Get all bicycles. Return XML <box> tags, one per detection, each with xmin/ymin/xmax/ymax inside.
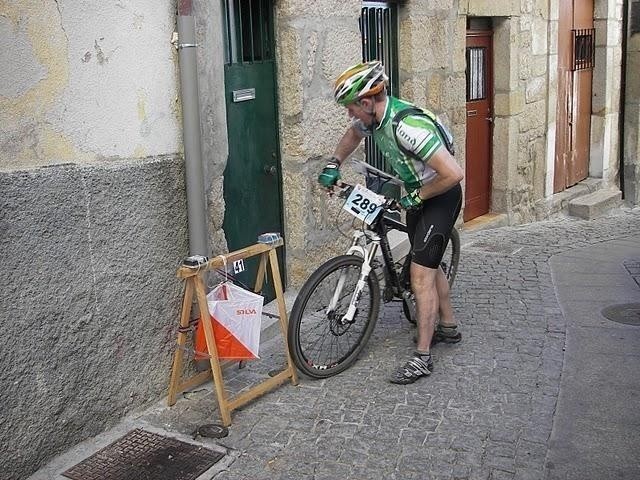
<box><xmin>284</xmin><ymin>172</ymin><xmax>466</xmax><ymax>375</ymax></box>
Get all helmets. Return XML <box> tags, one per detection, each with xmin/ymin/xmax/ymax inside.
<box><xmin>333</xmin><ymin>60</ymin><xmax>390</xmax><ymax>106</ymax></box>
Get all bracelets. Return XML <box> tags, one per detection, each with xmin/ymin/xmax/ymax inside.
<box><xmin>330</xmin><ymin>157</ymin><xmax>341</xmax><ymax>167</ymax></box>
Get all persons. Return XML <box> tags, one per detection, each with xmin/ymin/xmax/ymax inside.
<box><xmin>316</xmin><ymin>59</ymin><xmax>465</xmax><ymax>386</ymax></box>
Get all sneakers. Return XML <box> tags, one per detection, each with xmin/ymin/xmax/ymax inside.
<box><xmin>390</xmin><ymin>351</ymin><xmax>434</xmax><ymax>385</ymax></box>
<box><xmin>413</xmin><ymin>323</ymin><xmax>462</xmax><ymax>347</ymax></box>
